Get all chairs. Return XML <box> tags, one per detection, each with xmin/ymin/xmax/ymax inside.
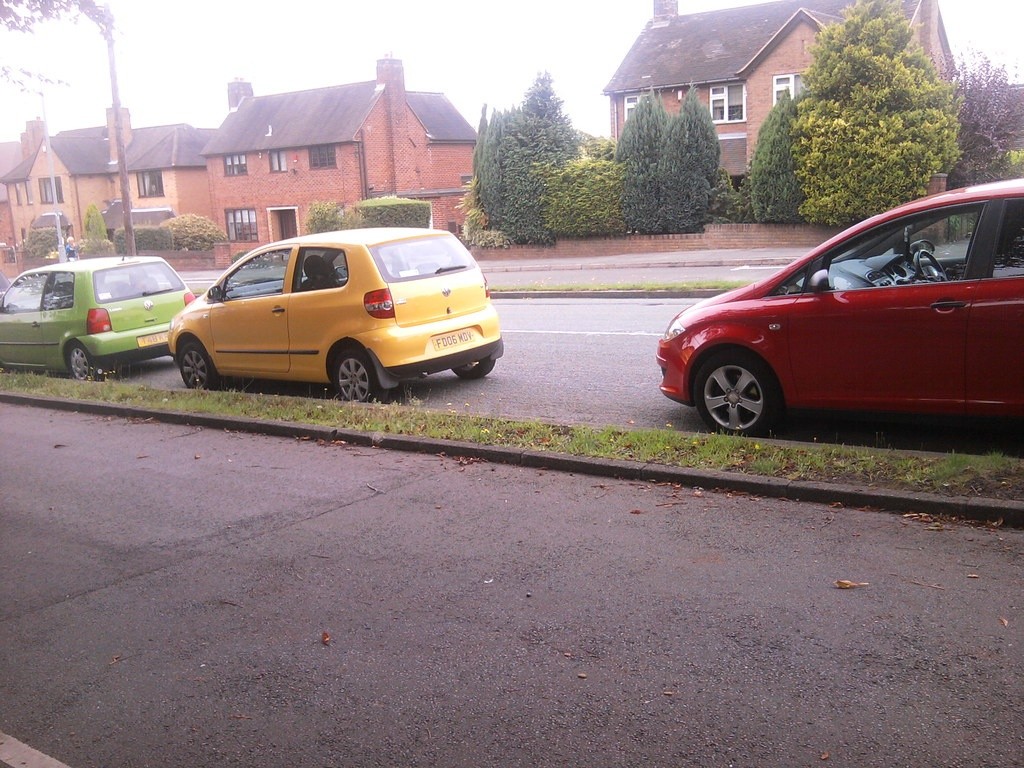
<box><xmin>302</xmin><ymin>255</ymin><xmax>333</xmax><ymax>291</ymax></box>
<box><xmin>51</xmin><ymin>281</ymin><xmax>74</xmax><ymax>309</ymax></box>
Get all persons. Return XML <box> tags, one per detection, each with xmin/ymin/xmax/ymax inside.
<box><xmin>65</xmin><ymin>236</ymin><xmax>80</xmax><ymax>263</ymax></box>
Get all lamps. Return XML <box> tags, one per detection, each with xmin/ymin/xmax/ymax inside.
<box><xmin>290</xmin><ymin>169</ymin><xmax>297</xmax><ymax>175</ymax></box>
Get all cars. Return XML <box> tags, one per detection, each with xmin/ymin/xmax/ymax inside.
<box><xmin>656</xmin><ymin>175</ymin><xmax>1024</xmax><ymax>434</ymax></box>
<box><xmin>0</xmin><ymin>254</ymin><xmax>195</xmax><ymax>379</ymax></box>
<box><xmin>166</xmin><ymin>227</ymin><xmax>504</xmax><ymax>403</ymax></box>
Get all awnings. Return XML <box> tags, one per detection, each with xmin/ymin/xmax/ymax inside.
<box><xmin>32</xmin><ymin>212</ymin><xmax>73</xmax><ymax>228</ymax></box>
<box><xmin>90</xmin><ymin>199</ymin><xmax>176</xmax><ymax>230</ymax></box>
